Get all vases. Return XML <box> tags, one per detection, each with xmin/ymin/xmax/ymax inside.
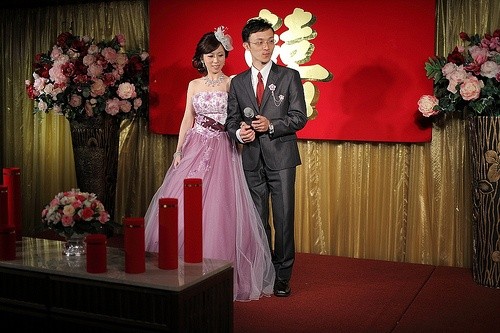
<box><xmin>467</xmin><ymin>112</ymin><xmax>500</xmax><ymax>289</ymax></box>
<box><xmin>68</xmin><ymin>112</ymin><xmax>119</xmax><ymax>221</ymax></box>
<box><xmin>59</xmin><ymin>231</ymin><xmax>88</xmax><ymax>256</ymax></box>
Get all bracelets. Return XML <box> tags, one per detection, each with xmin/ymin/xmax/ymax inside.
<box><xmin>174</xmin><ymin>151</ymin><xmax>182</xmax><ymax>157</ymax></box>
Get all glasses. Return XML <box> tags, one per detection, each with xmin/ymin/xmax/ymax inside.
<box><xmin>248</xmin><ymin>38</ymin><xmax>276</xmax><ymax>46</ymax></box>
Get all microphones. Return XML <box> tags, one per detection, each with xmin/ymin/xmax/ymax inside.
<box><xmin>243</xmin><ymin>107</ymin><xmax>257</xmax><ymax>121</ymax></box>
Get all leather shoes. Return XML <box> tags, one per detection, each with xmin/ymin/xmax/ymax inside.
<box><xmin>274</xmin><ymin>283</ymin><xmax>291</xmax><ymax>297</ymax></box>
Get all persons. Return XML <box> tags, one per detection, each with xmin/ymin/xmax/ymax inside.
<box><xmin>227</xmin><ymin>19</ymin><xmax>306</xmax><ymax>296</ymax></box>
<box><xmin>142</xmin><ymin>27</ymin><xmax>277</xmax><ymax>303</ymax></box>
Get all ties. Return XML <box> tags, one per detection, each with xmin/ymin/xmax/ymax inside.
<box><xmin>256</xmin><ymin>72</ymin><xmax>264</xmax><ymax>109</ymax></box>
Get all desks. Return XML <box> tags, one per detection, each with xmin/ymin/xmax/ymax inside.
<box><xmin>0</xmin><ymin>237</ymin><xmax>234</xmax><ymax>333</ymax></box>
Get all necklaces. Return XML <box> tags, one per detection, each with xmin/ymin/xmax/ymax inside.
<box><xmin>203</xmin><ymin>71</ymin><xmax>224</xmax><ymax>87</ymax></box>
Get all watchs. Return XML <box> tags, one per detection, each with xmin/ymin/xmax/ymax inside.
<box><xmin>268</xmin><ymin>122</ymin><xmax>274</xmax><ymax>134</ymax></box>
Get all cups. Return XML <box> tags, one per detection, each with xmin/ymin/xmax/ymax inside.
<box><xmin>0</xmin><ymin>167</ymin><xmax>22</xmax><ymax>261</ymax></box>
<box><xmin>184</xmin><ymin>178</ymin><xmax>202</xmax><ymax>263</ymax></box>
<box><xmin>86</xmin><ymin>234</ymin><xmax>108</xmax><ymax>273</ymax></box>
<box><xmin>125</xmin><ymin>217</ymin><xmax>146</xmax><ymax>273</ymax></box>
<box><xmin>158</xmin><ymin>198</ymin><xmax>178</xmax><ymax>269</ymax></box>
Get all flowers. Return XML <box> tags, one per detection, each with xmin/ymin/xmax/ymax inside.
<box><xmin>25</xmin><ymin>32</ymin><xmax>148</xmax><ymax>123</ymax></box>
<box><xmin>417</xmin><ymin>29</ymin><xmax>500</xmax><ymax>120</ymax></box>
<box><xmin>41</xmin><ymin>189</ymin><xmax>114</xmax><ymax>238</ymax></box>
